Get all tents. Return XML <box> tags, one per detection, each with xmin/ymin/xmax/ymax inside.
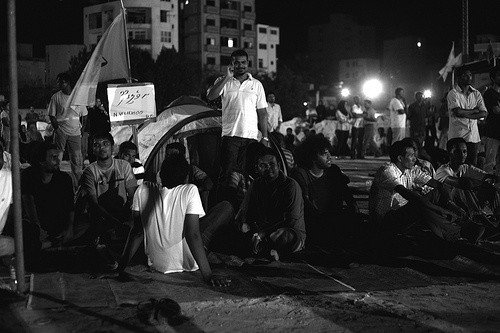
<box><xmin>133</xmin><ymin>106</ymin><xmax>222</xmax><ymax>183</ymax></box>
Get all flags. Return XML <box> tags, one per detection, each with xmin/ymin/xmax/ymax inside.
<box><xmin>65</xmin><ymin>9</ymin><xmax>129</xmax><ymax>117</ymax></box>
<box><xmin>438</xmin><ymin>46</ymin><xmax>462</xmax><ymax>84</ymax></box>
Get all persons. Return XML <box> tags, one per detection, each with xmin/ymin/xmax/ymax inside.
<box><xmin>207</xmin><ymin>50</ymin><xmax>269</xmax><ymax>204</ymax></box>
<box><xmin>0</xmin><ymin>67</ymin><xmax>500</xmax><ymax>288</ymax></box>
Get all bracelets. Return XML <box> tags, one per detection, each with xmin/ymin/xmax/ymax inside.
<box><xmin>261</xmin><ymin>137</ymin><xmax>269</xmax><ymax>142</ymax></box>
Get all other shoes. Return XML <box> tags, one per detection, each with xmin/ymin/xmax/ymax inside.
<box><xmin>136</xmin><ymin>297</ymin><xmax>187</xmax><ymax>326</ymax></box>
